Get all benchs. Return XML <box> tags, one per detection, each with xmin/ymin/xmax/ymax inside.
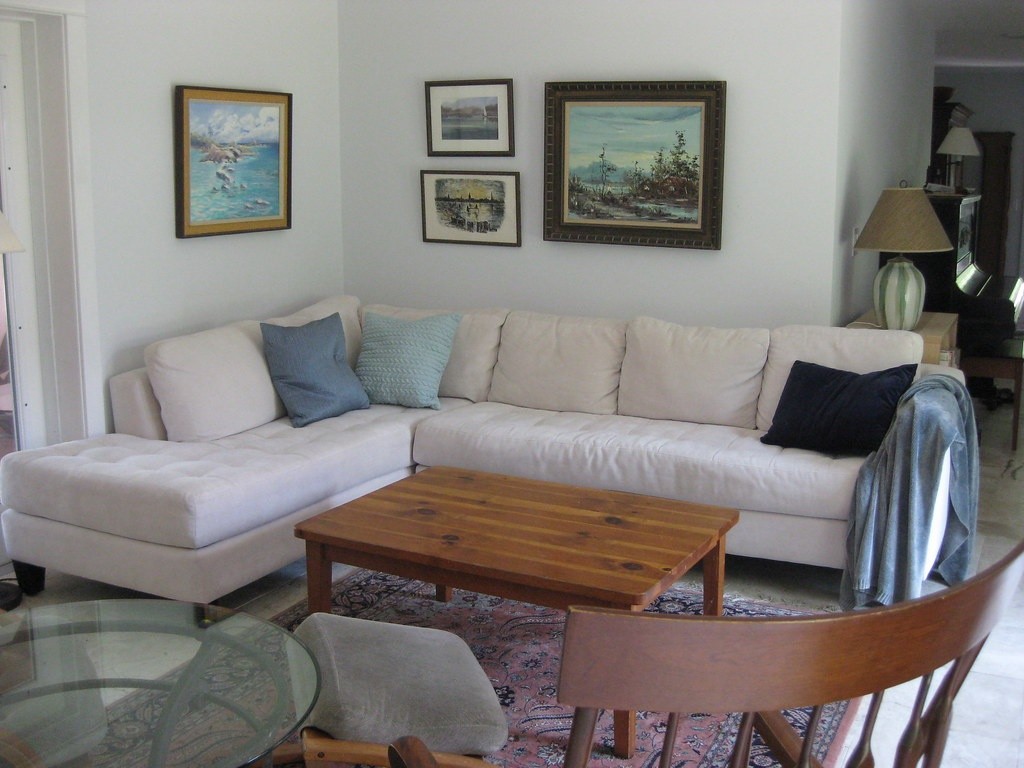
<box><xmin>961</xmin><ymin>331</ymin><xmax>1024</xmax><ymax>450</ymax></box>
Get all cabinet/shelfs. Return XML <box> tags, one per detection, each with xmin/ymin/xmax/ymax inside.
<box><xmin>849</xmin><ymin>308</ymin><xmax>958</xmax><ymax>365</ymax></box>
<box><xmin>936</xmin><ymin>103</ymin><xmax>976</xmax><ymax>193</ymax></box>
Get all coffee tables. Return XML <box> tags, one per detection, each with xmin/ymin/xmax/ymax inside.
<box><xmin>295</xmin><ymin>465</ymin><xmax>739</xmax><ymax>758</ymax></box>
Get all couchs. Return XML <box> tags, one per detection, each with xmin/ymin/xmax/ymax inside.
<box><xmin>2</xmin><ymin>295</ymin><xmax>967</xmax><ymax>626</ymax></box>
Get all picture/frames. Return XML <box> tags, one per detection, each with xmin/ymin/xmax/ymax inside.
<box><xmin>543</xmin><ymin>81</ymin><xmax>727</xmax><ymax>250</ymax></box>
<box><xmin>420</xmin><ymin>170</ymin><xmax>521</xmax><ymax>247</ymax></box>
<box><xmin>175</xmin><ymin>85</ymin><xmax>293</xmax><ymax>238</ymax></box>
<box><xmin>425</xmin><ymin>78</ymin><xmax>515</xmax><ymax>157</ymax></box>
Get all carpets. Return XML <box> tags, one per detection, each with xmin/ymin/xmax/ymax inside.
<box><xmin>0</xmin><ymin>569</ymin><xmax>860</xmax><ymax>768</ymax></box>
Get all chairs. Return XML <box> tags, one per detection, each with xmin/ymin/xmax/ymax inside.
<box><xmin>287</xmin><ymin>612</ymin><xmax>508</xmax><ymax>768</ymax></box>
<box><xmin>556</xmin><ymin>538</ymin><xmax>1024</xmax><ymax>768</ymax></box>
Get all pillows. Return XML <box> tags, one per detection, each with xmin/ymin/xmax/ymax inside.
<box><xmin>260</xmin><ymin>312</ymin><xmax>370</xmax><ymax>427</ymax></box>
<box><xmin>356</xmin><ymin>311</ymin><xmax>461</xmax><ymax>410</ymax></box>
<box><xmin>760</xmin><ymin>360</ymin><xmax>918</xmax><ymax>457</ymax></box>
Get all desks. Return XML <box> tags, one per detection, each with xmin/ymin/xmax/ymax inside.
<box><xmin>0</xmin><ymin>600</ymin><xmax>321</xmax><ymax>768</ymax></box>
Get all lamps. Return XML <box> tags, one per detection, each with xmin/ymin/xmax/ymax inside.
<box><xmin>930</xmin><ymin>126</ymin><xmax>981</xmax><ymax>170</ymax></box>
<box><xmin>854</xmin><ymin>180</ymin><xmax>954</xmax><ymax>331</ymax></box>
